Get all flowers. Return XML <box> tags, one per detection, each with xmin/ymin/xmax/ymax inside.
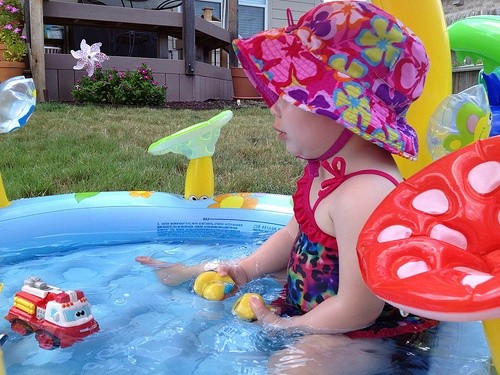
<box><xmin>0</xmin><ymin>0</ymin><xmax>28</xmax><ymax>62</ymax></box>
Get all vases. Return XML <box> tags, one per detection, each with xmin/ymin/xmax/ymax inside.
<box><xmin>231</xmin><ymin>68</ymin><xmax>257</xmax><ymax>97</ymax></box>
<box><xmin>0</xmin><ymin>44</ymin><xmax>25</xmax><ymax>81</ymax></box>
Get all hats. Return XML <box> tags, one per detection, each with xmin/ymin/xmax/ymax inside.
<box><xmin>231</xmin><ymin>0</ymin><xmax>430</xmax><ymax>164</ymax></box>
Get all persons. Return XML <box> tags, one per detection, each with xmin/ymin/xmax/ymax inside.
<box><xmin>131</xmin><ymin>1</ymin><xmax>442</xmax><ymax>375</ymax></box>
